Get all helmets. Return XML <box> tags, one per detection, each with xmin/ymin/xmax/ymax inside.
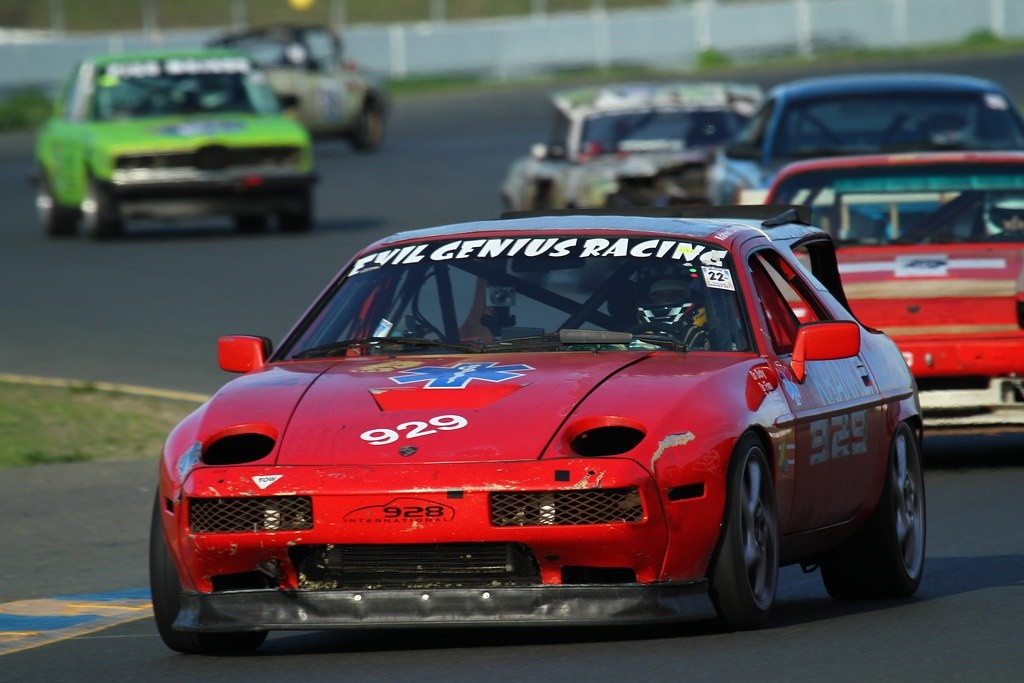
<box><xmin>927</xmin><ymin>101</ymin><xmax>970</xmax><ymax>147</ymax></box>
<box><xmin>981</xmin><ymin>189</ymin><xmax>1024</xmax><ymax>237</ymax></box>
<box><xmin>632</xmin><ymin>257</ymin><xmax>711</xmax><ymax>333</ymax></box>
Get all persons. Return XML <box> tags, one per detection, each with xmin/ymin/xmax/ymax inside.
<box><xmin>623</xmin><ymin>273</ymin><xmax>716</xmax><ymax>351</ymax></box>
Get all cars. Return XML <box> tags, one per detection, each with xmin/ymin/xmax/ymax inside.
<box><xmin>206</xmin><ymin>23</ymin><xmax>388</xmax><ymax>150</ymax></box>
<box><xmin>704</xmin><ymin>75</ymin><xmax>1024</xmax><ymax>206</ymax></box>
<box><xmin>36</xmin><ymin>47</ymin><xmax>318</xmax><ymax>237</ymax></box>
<box><xmin>767</xmin><ymin>147</ymin><xmax>1024</xmax><ymax>437</ymax></box>
<box><xmin>498</xmin><ymin>81</ymin><xmax>766</xmax><ymax>219</ymax></box>
<box><xmin>149</xmin><ymin>212</ymin><xmax>931</xmax><ymax>649</ymax></box>
